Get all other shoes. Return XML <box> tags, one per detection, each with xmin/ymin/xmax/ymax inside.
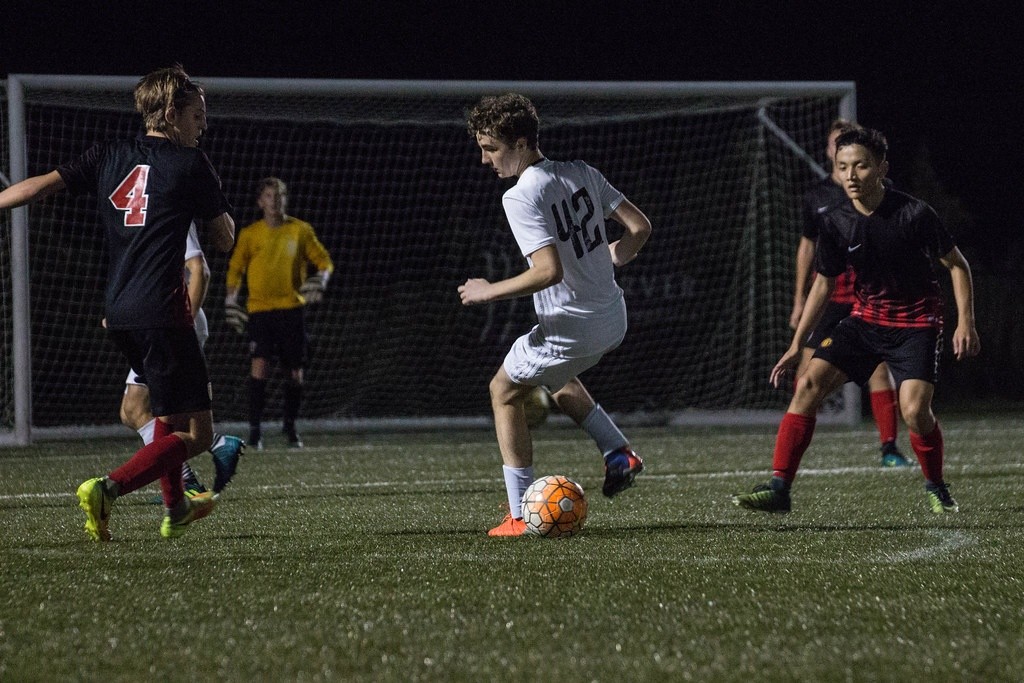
<box><xmin>247</xmin><ymin>437</ymin><xmax>262</xmax><ymax>451</ymax></box>
<box><xmin>283</xmin><ymin>426</ymin><xmax>304</xmax><ymax>448</ymax></box>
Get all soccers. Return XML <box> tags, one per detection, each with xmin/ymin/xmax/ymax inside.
<box><xmin>520</xmin><ymin>475</ymin><xmax>588</xmax><ymax>538</ymax></box>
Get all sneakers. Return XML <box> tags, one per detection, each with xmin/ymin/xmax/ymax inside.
<box><xmin>488</xmin><ymin>513</ymin><xmax>527</xmax><ymax>536</ymax></box>
<box><xmin>603</xmin><ymin>449</ymin><xmax>643</xmax><ymax>498</ymax></box>
<box><xmin>156</xmin><ymin>480</ymin><xmax>206</xmax><ymax>504</ymax></box>
<box><xmin>731</xmin><ymin>480</ymin><xmax>790</xmax><ymax>514</ymax></box>
<box><xmin>881</xmin><ymin>443</ymin><xmax>916</xmax><ymax>467</ymax></box>
<box><xmin>161</xmin><ymin>492</ymin><xmax>219</xmax><ymax>538</ymax></box>
<box><xmin>76</xmin><ymin>478</ymin><xmax>114</xmax><ymax>542</ymax></box>
<box><xmin>923</xmin><ymin>481</ymin><xmax>959</xmax><ymax>514</ymax></box>
<box><xmin>212</xmin><ymin>435</ymin><xmax>245</xmax><ymax>494</ymax></box>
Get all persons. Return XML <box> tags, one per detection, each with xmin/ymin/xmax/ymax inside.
<box><xmin>121</xmin><ymin>222</ymin><xmax>244</xmax><ymax>504</ymax></box>
<box><xmin>0</xmin><ymin>66</ymin><xmax>235</xmax><ymax>543</ymax></box>
<box><xmin>732</xmin><ymin>118</ymin><xmax>979</xmax><ymax>514</ymax></box>
<box><xmin>458</xmin><ymin>92</ymin><xmax>651</xmax><ymax>537</ymax></box>
<box><xmin>225</xmin><ymin>178</ymin><xmax>334</xmax><ymax>449</ymax></box>
